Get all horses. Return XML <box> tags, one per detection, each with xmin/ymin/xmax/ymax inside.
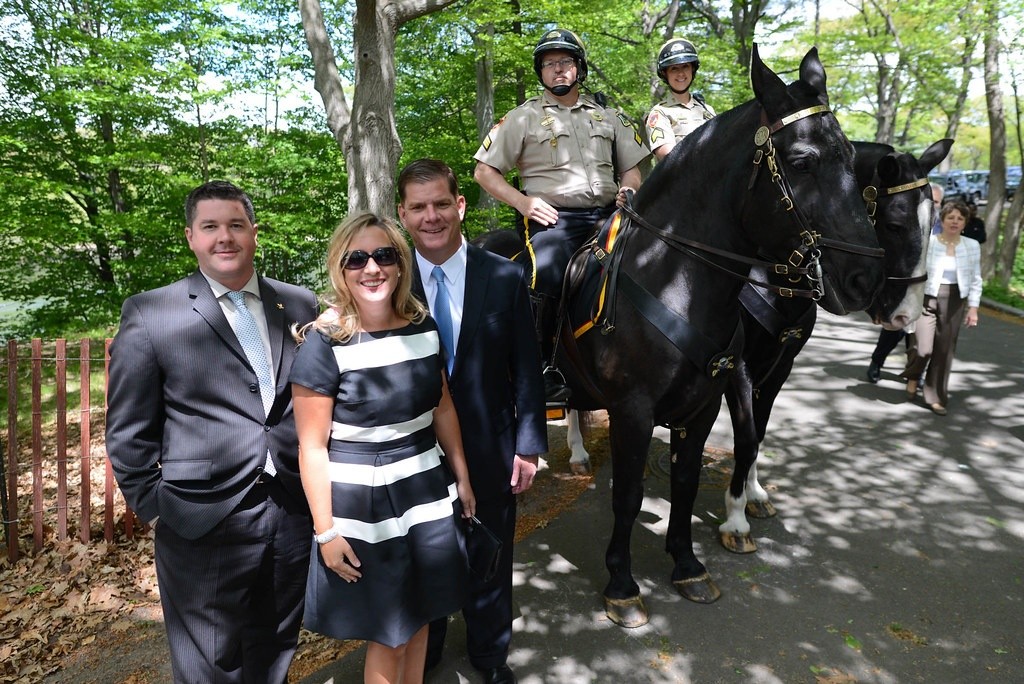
<box><xmin>471</xmin><ymin>41</ymin><xmax>955</xmax><ymax>629</ymax></box>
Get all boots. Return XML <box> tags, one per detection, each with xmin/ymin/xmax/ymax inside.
<box><xmin>528</xmin><ymin>286</ymin><xmax>571</xmax><ymax>400</ymax></box>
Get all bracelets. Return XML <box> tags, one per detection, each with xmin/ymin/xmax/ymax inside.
<box><xmin>313</xmin><ymin>525</ymin><xmax>338</xmax><ymax>544</ymax></box>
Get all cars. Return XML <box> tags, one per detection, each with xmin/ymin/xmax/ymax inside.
<box><xmin>951</xmin><ymin>169</ymin><xmax>990</xmax><ymax>204</ymax></box>
<box><xmin>1005</xmin><ymin>167</ymin><xmax>1022</xmax><ymax>199</ymax></box>
<box><xmin>927</xmin><ymin>173</ymin><xmax>964</xmax><ymax>207</ymax></box>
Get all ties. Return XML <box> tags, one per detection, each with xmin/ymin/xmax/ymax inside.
<box><xmin>226</xmin><ymin>291</ymin><xmax>278</xmax><ymax>476</ymax></box>
<box><xmin>432</xmin><ymin>266</ymin><xmax>456</xmax><ymax>380</ymax></box>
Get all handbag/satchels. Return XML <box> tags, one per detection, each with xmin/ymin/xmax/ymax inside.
<box><xmin>453</xmin><ymin>504</ymin><xmax>502</xmax><ymax>582</ymax></box>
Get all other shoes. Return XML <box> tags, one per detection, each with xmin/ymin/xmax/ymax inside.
<box><xmin>868</xmin><ymin>360</ymin><xmax>879</xmax><ymax>382</ymax></box>
<box><xmin>906</xmin><ymin>379</ymin><xmax>918</xmax><ymax>399</ymax></box>
<box><xmin>927</xmin><ymin>402</ymin><xmax>947</xmax><ymax>415</ymax></box>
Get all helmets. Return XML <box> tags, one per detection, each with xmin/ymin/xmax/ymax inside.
<box><xmin>532</xmin><ymin>28</ymin><xmax>589</xmax><ymax>77</ymax></box>
<box><xmin>657</xmin><ymin>41</ymin><xmax>700</xmax><ymax>78</ymax></box>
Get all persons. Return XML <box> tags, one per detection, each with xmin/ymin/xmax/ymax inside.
<box><xmin>473</xmin><ymin>29</ymin><xmax>649</xmax><ymax>402</ymax></box>
<box><xmin>105</xmin><ymin>180</ymin><xmax>319</xmax><ymax>684</ymax></box>
<box><xmin>897</xmin><ymin>198</ymin><xmax>987</xmax><ymax>416</ymax></box>
<box><xmin>645</xmin><ymin>38</ymin><xmax>717</xmax><ymax>160</ymax></box>
<box><xmin>867</xmin><ymin>182</ymin><xmax>944</xmax><ymax>388</ymax></box>
<box><xmin>287</xmin><ymin>214</ymin><xmax>475</xmax><ymax>684</ymax></box>
<box><xmin>397</xmin><ymin>159</ymin><xmax>548</xmax><ymax>684</ymax></box>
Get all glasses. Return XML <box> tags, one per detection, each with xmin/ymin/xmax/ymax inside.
<box><xmin>539</xmin><ymin>57</ymin><xmax>576</xmax><ymax>68</ymax></box>
<box><xmin>341</xmin><ymin>247</ymin><xmax>399</xmax><ymax>270</ymax></box>
